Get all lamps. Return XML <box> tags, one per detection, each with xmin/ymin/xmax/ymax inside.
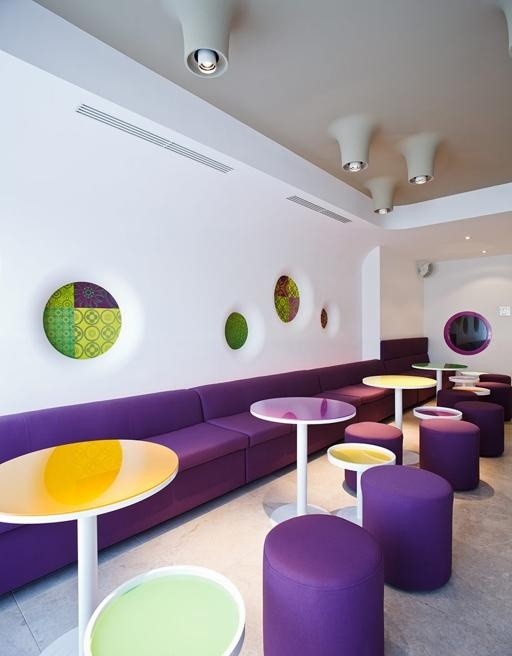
<box><xmin>415</xmin><ymin>176</ymin><xmax>427</xmax><ymax>184</ymax></box>
<box><xmin>349</xmin><ymin>162</ymin><xmax>362</xmax><ymax>173</ymax></box>
<box><xmin>379</xmin><ymin>208</ymin><xmax>387</xmax><ymax>215</ymax></box>
<box><xmin>197</xmin><ymin>50</ymin><xmax>217</xmax><ymax>74</ymax></box>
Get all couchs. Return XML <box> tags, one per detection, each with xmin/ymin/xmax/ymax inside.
<box><xmin>191</xmin><ymin>370</ymin><xmax>362</xmax><ymax>485</ymax></box>
<box><xmin>0</xmin><ymin>389</ymin><xmax>250</xmax><ymax>597</ymax></box>
<box><xmin>381</xmin><ymin>337</ymin><xmax>455</xmax><ymax>406</ymax></box>
<box><xmin>314</xmin><ymin>359</ymin><xmax>419</xmax><ymax>421</ymax></box>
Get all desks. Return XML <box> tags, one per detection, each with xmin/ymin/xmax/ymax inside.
<box><xmin>363</xmin><ymin>375</ymin><xmax>439</xmax><ymax>467</ymax></box>
<box><xmin>0</xmin><ymin>439</ymin><xmax>180</xmax><ymax>656</ymax></box>
<box><xmin>413</xmin><ymin>405</ymin><xmax>463</xmax><ymax>421</ymax></box>
<box><xmin>412</xmin><ymin>363</ymin><xmax>468</xmax><ymax>393</ymax></box>
<box><xmin>83</xmin><ymin>564</ymin><xmax>246</xmax><ymax>656</ymax></box>
<box><xmin>327</xmin><ymin>442</ymin><xmax>396</xmax><ymax>526</ymax></box>
<box><xmin>449</xmin><ymin>376</ymin><xmax>477</xmax><ymax>386</ymax></box>
<box><xmin>251</xmin><ymin>397</ymin><xmax>357</xmax><ymax>528</ymax></box>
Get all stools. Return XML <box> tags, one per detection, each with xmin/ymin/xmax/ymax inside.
<box><xmin>476</xmin><ymin>382</ymin><xmax>512</xmax><ymax>421</ymax></box>
<box><xmin>479</xmin><ymin>374</ymin><xmax>511</xmax><ymax>384</ymax></box>
<box><xmin>455</xmin><ymin>400</ymin><xmax>504</xmax><ymax>458</ymax></box>
<box><xmin>437</xmin><ymin>390</ymin><xmax>479</xmax><ymax>409</ymax></box>
<box><xmin>361</xmin><ymin>465</ymin><xmax>454</xmax><ymax>591</ymax></box>
<box><xmin>263</xmin><ymin>513</ymin><xmax>385</xmax><ymax>655</ymax></box>
<box><xmin>419</xmin><ymin>418</ymin><xmax>481</xmax><ymax>490</ymax></box>
<box><xmin>345</xmin><ymin>422</ymin><xmax>403</xmax><ymax>491</ymax></box>
